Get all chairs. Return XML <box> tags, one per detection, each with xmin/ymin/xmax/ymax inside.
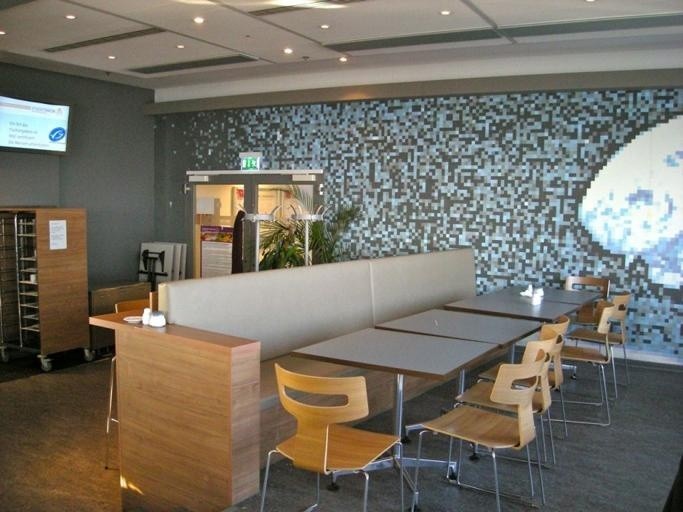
<box><xmin>104</xmin><ymin>299</ymin><xmax>150</xmax><ymax>470</ymax></box>
<box><xmin>261</xmin><ymin>276</ymin><xmax>632</xmax><ymax>512</ymax></box>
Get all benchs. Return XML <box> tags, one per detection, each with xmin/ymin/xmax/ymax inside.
<box><xmin>156</xmin><ymin>247</ymin><xmax>505</xmax><ymax>469</ymax></box>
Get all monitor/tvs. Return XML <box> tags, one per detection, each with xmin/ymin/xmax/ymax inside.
<box><xmin>0</xmin><ymin>91</ymin><xmax>74</xmax><ymax>156</ymax></box>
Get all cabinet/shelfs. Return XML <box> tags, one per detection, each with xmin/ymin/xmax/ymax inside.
<box><xmin>0</xmin><ymin>205</ymin><xmax>93</xmax><ymax>371</ymax></box>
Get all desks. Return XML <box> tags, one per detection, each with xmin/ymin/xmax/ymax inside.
<box><xmin>260</xmin><ymin>275</ymin><xmax>633</xmax><ymax>511</ymax></box>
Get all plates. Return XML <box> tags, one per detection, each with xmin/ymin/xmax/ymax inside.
<box><xmin>122</xmin><ymin>316</ymin><xmax>142</xmax><ymax>324</ymax></box>
